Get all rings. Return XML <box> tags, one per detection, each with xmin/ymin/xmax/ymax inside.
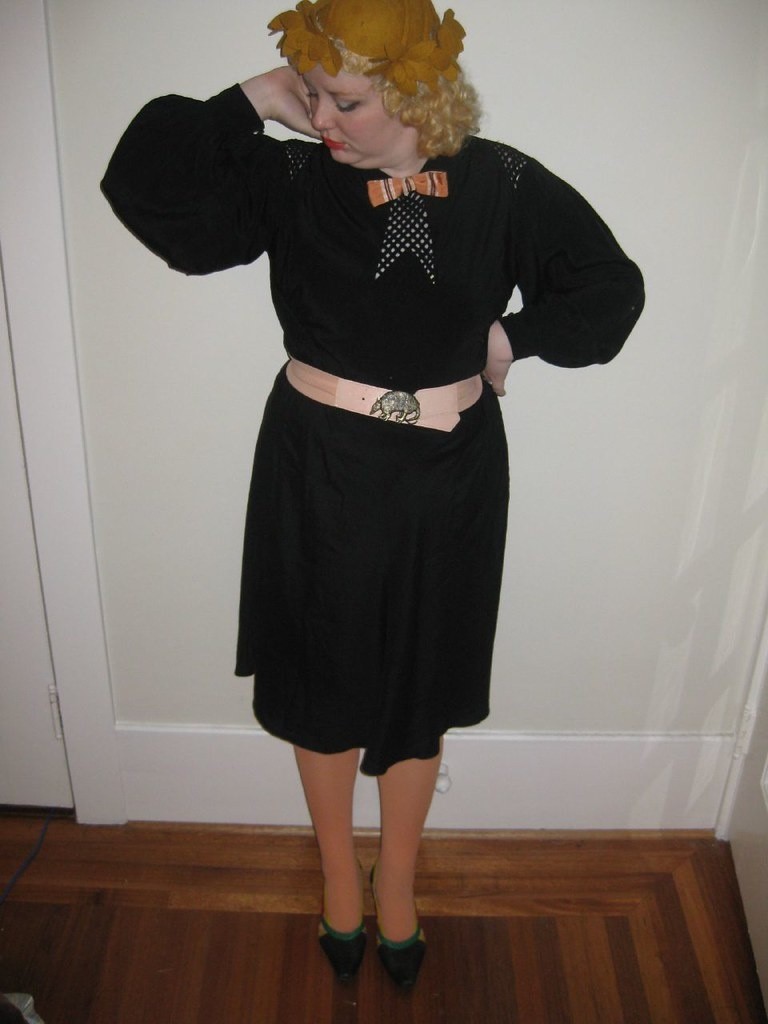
<box><xmin>487</xmin><ymin>381</ymin><xmax>492</xmax><ymax>384</ymax></box>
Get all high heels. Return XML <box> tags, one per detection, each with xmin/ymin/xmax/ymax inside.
<box><xmin>370</xmin><ymin>861</ymin><xmax>425</xmax><ymax>995</ymax></box>
<box><xmin>318</xmin><ymin>858</ymin><xmax>367</xmax><ymax>986</ymax></box>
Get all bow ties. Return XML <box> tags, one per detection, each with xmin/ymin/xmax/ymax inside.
<box><xmin>366</xmin><ymin>170</ymin><xmax>449</xmax><ymax>286</ymax></box>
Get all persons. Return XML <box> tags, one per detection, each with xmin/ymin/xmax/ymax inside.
<box><xmin>96</xmin><ymin>0</ymin><xmax>646</xmax><ymax>988</ymax></box>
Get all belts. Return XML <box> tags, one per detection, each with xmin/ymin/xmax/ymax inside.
<box><xmin>285</xmin><ymin>351</ymin><xmax>483</xmax><ymax>432</ymax></box>
